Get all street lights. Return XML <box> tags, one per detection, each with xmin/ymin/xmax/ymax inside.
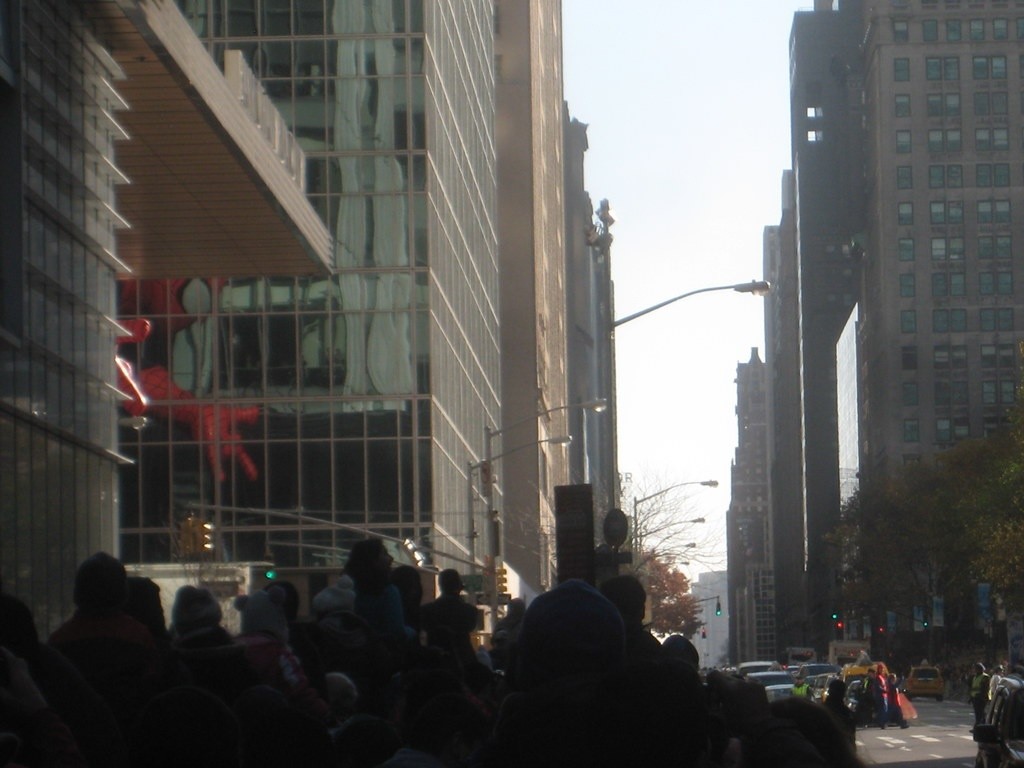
<box><xmin>633</xmin><ymin>477</ymin><xmax>720</xmax><ymax>581</ymax></box>
<box><xmin>481</xmin><ymin>396</ymin><xmax>607</xmax><ymax>622</ymax></box>
<box><xmin>604</xmin><ymin>202</ymin><xmax>770</xmax><ymax>506</ymax></box>
<box><xmin>466</xmin><ymin>435</ymin><xmax>572</xmax><ymax>607</ymax></box>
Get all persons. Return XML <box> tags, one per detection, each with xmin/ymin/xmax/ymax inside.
<box><xmin>987</xmin><ymin>664</ymin><xmax>1005</xmax><ymax>700</ymax></box>
<box><xmin>1</xmin><ymin>533</ymin><xmax>862</xmax><ymax>767</ymax></box>
<box><xmin>872</xmin><ymin>664</ymin><xmax>890</xmax><ymax>727</ymax></box>
<box><xmin>966</xmin><ymin>661</ymin><xmax>990</xmax><ymax>733</ymax></box>
<box><xmin>854</xmin><ymin>668</ymin><xmax>877</xmax><ymax>724</ymax></box>
<box><xmin>881</xmin><ymin>673</ymin><xmax>910</xmax><ymax>729</ymax></box>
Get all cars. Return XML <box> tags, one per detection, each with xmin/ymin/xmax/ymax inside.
<box><xmin>716</xmin><ymin>639</ymin><xmax>899</xmax><ymax>726</ymax></box>
<box><xmin>972</xmin><ymin>665</ymin><xmax>1024</xmax><ymax>768</ymax></box>
<box><xmin>904</xmin><ymin>666</ymin><xmax>945</xmax><ymax>701</ymax></box>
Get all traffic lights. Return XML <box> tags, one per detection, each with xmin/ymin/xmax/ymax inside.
<box><xmin>878</xmin><ymin>625</ymin><xmax>884</xmax><ymax>633</ymax></box>
<box><xmin>832</xmin><ymin>608</ymin><xmax>843</xmax><ymax>631</ymax></box>
<box><xmin>264</xmin><ymin>555</ymin><xmax>276</xmax><ymax>579</ymax></box>
<box><xmin>716</xmin><ymin>603</ymin><xmax>720</xmax><ymax>616</ymax></box>
<box><xmin>923</xmin><ymin>616</ymin><xmax>928</xmax><ymax>628</ymax></box>
<box><xmin>702</xmin><ymin>628</ymin><xmax>707</xmax><ymax>639</ymax></box>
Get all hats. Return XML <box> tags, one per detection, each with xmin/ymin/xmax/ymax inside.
<box><xmin>795</xmin><ymin>674</ymin><xmax>804</xmax><ymax>680</ymax></box>
<box><xmin>265</xmin><ymin>582</ymin><xmax>300</xmax><ymax>606</ymax></box>
<box><xmin>438</xmin><ymin>569</ymin><xmax>464</xmax><ymax>586</ymax></box>
<box><xmin>126</xmin><ymin>578</ymin><xmax>163</xmax><ymax>614</ymax></box>
<box><xmin>74</xmin><ymin>553</ymin><xmax>129</xmax><ymax>604</ymax></box>
<box><xmin>868</xmin><ymin>668</ymin><xmax>874</xmax><ymax>672</ymax></box>
<box><xmin>601</xmin><ymin>573</ymin><xmax>646</xmax><ymax>602</ymax></box>
<box><xmin>520</xmin><ymin>578</ymin><xmax>625</xmax><ymax>666</ymax></box>
<box><xmin>977</xmin><ymin>662</ymin><xmax>985</xmax><ymax>671</ymax></box>
